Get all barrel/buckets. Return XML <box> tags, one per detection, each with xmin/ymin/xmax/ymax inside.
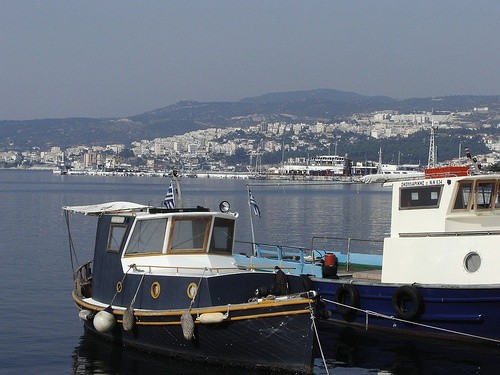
<box><xmin>324</xmin><ymin>253</ymin><xmax>335</xmax><ymax>267</ymax></box>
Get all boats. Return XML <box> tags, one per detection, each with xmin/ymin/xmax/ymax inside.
<box><xmin>228</xmin><ymin>165</ymin><xmax>500</xmax><ymax>346</ymax></box>
<box><xmin>61</xmin><ymin>200</ymin><xmax>320</xmax><ymax>375</ymax></box>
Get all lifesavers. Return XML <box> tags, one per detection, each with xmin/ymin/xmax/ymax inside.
<box><xmin>392</xmin><ymin>286</ymin><xmax>424</xmax><ymax>321</ymax></box>
<box><xmin>336</xmin><ymin>284</ymin><xmax>361</xmax><ymax>322</ymax></box>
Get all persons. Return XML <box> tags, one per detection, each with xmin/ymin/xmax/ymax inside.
<box><xmin>273</xmin><ymin>266</ymin><xmax>289</xmax><ymax>296</ymax></box>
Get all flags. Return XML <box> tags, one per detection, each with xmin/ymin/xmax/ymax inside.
<box><xmin>248</xmin><ymin>189</ymin><xmax>261</xmax><ymax>217</ymax></box>
<box><xmin>163</xmin><ymin>181</ymin><xmax>175</xmax><ymax>210</ymax></box>
